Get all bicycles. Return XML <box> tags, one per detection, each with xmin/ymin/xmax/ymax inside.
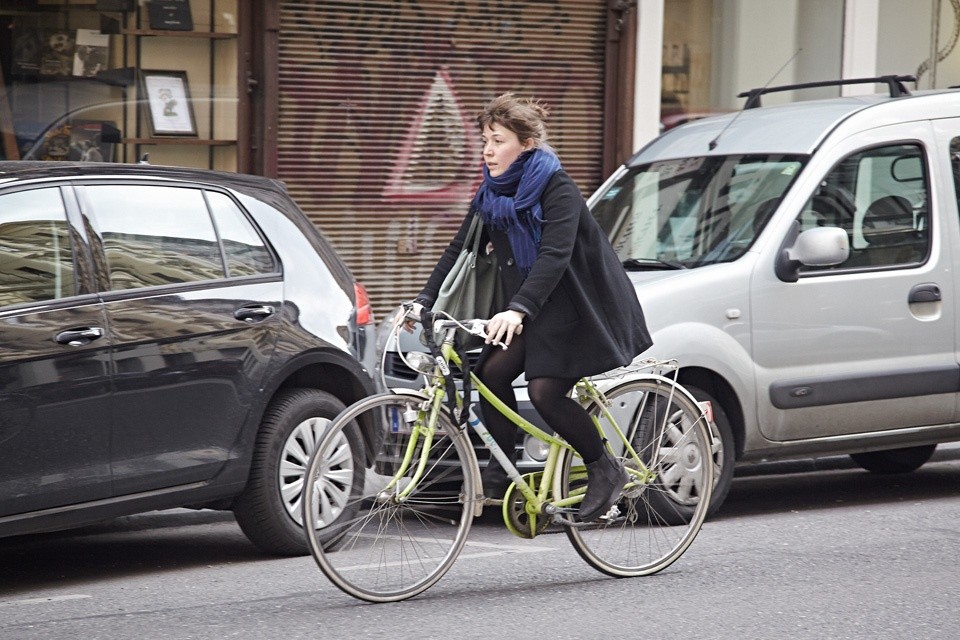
<box><xmin>301</xmin><ymin>308</ymin><xmax>724</xmax><ymax>604</ymax></box>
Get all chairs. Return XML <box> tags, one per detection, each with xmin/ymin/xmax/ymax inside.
<box><xmin>752</xmin><ymin>197</ymin><xmax>803</xmax><ymax>240</ymax></box>
<box><xmin>859</xmin><ymin>196</ymin><xmax>927</xmax><ymax>266</ymax></box>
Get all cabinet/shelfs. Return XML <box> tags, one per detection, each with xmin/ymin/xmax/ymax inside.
<box><xmin>0</xmin><ymin>0</ymin><xmax>241</xmax><ymax>172</ymax></box>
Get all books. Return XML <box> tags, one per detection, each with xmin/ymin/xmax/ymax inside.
<box><xmin>7</xmin><ymin>27</ymin><xmax>110</xmax><ymax>77</ymax></box>
<box><xmin>14</xmin><ymin>120</ymin><xmax>116</xmax><ymax>162</ymax></box>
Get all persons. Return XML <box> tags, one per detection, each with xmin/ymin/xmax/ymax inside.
<box><xmin>395</xmin><ymin>90</ymin><xmax>653</xmax><ymax>522</ymax></box>
<box><xmin>78</xmin><ymin>45</ymin><xmax>102</xmax><ymax>77</ymax></box>
<box><xmin>75</xmin><ymin>139</ymin><xmax>103</xmax><ymax>162</ymax></box>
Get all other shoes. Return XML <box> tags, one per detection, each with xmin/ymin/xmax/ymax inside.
<box><xmin>579</xmin><ymin>450</ymin><xmax>629</xmax><ymax>524</ymax></box>
<box><xmin>461</xmin><ymin>449</ymin><xmax>518</xmax><ymax>499</ymax></box>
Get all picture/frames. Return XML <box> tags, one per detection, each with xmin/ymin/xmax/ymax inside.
<box><xmin>136</xmin><ymin>67</ymin><xmax>199</xmax><ymax>139</ymax></box>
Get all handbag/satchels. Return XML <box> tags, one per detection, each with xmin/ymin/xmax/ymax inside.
<box><xmin>431</xmin><ymin>206</ymin><xmax>511</xmax><ymax>353</ymax></box>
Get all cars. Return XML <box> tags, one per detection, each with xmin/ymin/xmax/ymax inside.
<box><xmin>0</xmin><ymin>152</ymin><xmax>387</xmax><ymax>557</ymax></box>
<box><xmin>368</xmin><ymin>75</ymin><xmax>959</xmax><ymax>519</ymax></box>
